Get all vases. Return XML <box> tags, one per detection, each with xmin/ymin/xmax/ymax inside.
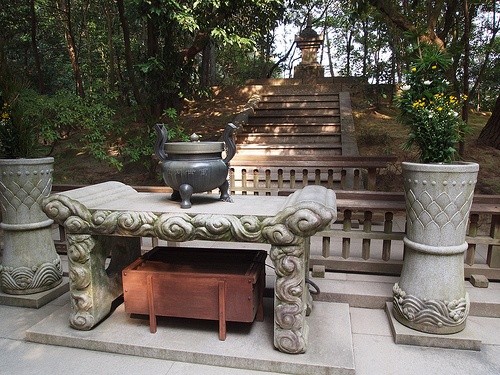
<box><xmin>392</xmin><ymin>160</ymin><xmax>479</xmax><ymax>335</ymax></box>
<box><xmin>0</xmin><ymin>157</ymin><xmax>62</xmax><ymax>296</ymax></box>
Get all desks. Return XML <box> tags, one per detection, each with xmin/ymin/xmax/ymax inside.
<box><xmin>40</xmin><ymin>180</ymin><xmax>337</xmax><ymax>354</ymax></box>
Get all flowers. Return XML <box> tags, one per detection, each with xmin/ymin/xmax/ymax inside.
<box><xmin>392</xmin><ymin>25</ymin><xmax>477</xmax><ymax>165</ymax></box>
<box><xmin>0</xmin><ymin>76</ymin><xmax>46</xmax><ymax>159</ymax></box>
